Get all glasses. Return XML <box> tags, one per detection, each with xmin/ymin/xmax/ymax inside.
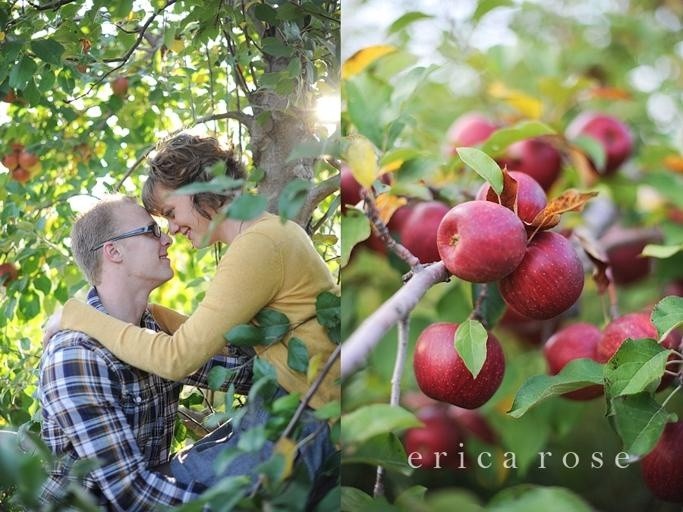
<box><xmin>92</xmin><ymin>225</ymin><xmax>162</xmax><ymax>251</ymax></box>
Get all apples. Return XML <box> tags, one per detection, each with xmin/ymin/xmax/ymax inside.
<box><xmin>371</xmin><ymin>171</ymin><xmax>393</xmax><ymax>192</ymax></box>
<box><xmin>74</xmin><ymin>143</ymin><xmax>90</xmax><ymax>160</ymax></box>
<box><xmin>0</xmin><ymin>264</ymin><xmax>18</xmax><ymax>285</ymax></box>
<box><xmin>364</xmin><ymin>231</ymin><xmax>386</xmax><ymax>253</ymax></box>
<box><xmin>76</xmin><ymin>60</ymin><xmax>87</xmax><ymax>73</ymax></box>
<box><xmin>340</xmin><ymin>167</ymin><xmax>361</xmax><ymax>205</ymax></box>
<box><xmin>10</xmin><ymin>143</ymin><xmax>23</xmax><ymax>155</ymax></box>
<box><xmin>571</xmin><ymin>113</ymin><xmax>633</xmax><ymax>177</ymax></box>
<box><xmin>15</xmin><ymin>94</ymin><xmax>28</xmax><ymax>106</ymax></box>
<box><xmin>642</xmin><ymin>420</ymin><xmax>683</xmax><ymax>500</ymax></box>
<box><xmin>609</xmin><ymin>243</ymin><xmax>651</xmax><ymax>286</ymax></box>
<box><xmin>13</xmin><ymin>165</ymin><xmax>29</xmax><ymax>182</ymax></box>
<box><xmin>402</xmin><ymin>390</ymin><xmax>442</xmax><ymax>418</ymax></box>
<box><xmin>3</xmin><ymin>88</ymin><xmax>15</xmax><ymax>102</ymax></box>
<box><xmin>79</xmin><ymin>39</ymin><xmax>91</xmax><ymax>52</ymax></box>
<box><xmin>436</xmin><ymin>201</ymin><xmax>527</xmax><ymax>284</ymax></box>
<box><xmin>544</xmin><ymin>323</ymin><xmax>606</xmax><ymax>402</ymax></box>
<box><xmin>661</xmin><ymin>278</ymin><xmax>682</xmax><ymax>301</ymax></box>
<box><xmin>499</xmin><ymin>230</ymin><xmax>585</xmax><ymax>322</ymax></box>
<box><xmin>645</xmin><ymin>303</ymin><xmax>680</xmax><ymax>392</ymax></box>
<box><xmin>404</xmin><ymin>404</ymin><xmax>463</xmax><ymax>471</ymax></box>
<box><xmin>18</xmin><ymin>149</ymin><xmax>40</xmax><ymax>169</ymax></box>
<box><xmin>414</xmin><ymin>321</ymin><xmax>506</xmax><ymax>409</ymax></box>
<box><xmin>374</xmin><ymin>191</ymin><xmax>410</xmax><ymax>232</ymax></box>
<box><xmin>110</xmin><ymin>77</ymin><xmax>128</xmax><ymax>94</ymax></box>
<box><xmin>400</xmin><ymin>200</ymin><xmax>450</xmax><ymax>265</ymax></box>
<box><xmin>477</xmin><ymin>170</ymin><xmax>549</xmax><ymax>239</ymax></box>
<box><xmin>2</xmin><ymin>154</ymin><xmax>20</xmax><ymax>171</ymax></box>
<box><xmin>444</xmin><ymin>113</ymin><xmax>495</xmax><ymax>158</ymax></box>
<box><xmin>450</xmin><ymin>404</ymin><xmax>499</xmax><ymax>446</ymax></box>
<box><xmin>597</xmin><ymin>313</ymin><xmax>657</xmax><ymax>365</ymax></box>
<box><xmin>499</xmin><ymin>140</ymin><xmax>562</xmax><ymax>189</ymax></box>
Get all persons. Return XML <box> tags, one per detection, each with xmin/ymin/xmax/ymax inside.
<box><xmin>41</xmin><ymin>199</ymin><xmax>255</xmax><ymax>511</ymax></box>
<box><xmin>41</xmin><ymin>134</ymin><xmax>340</xmax><ymax>509</ymax></box>
